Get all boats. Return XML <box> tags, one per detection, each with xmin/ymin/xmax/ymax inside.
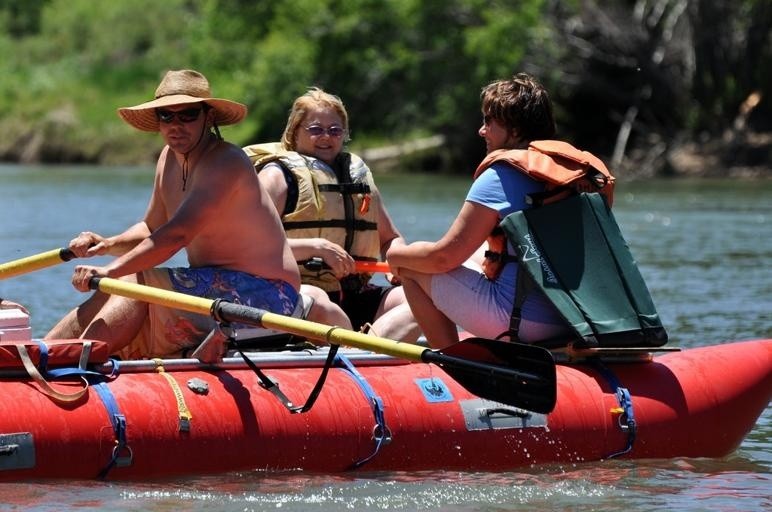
<box><xmin>0</xmin><ymin>260</ymin><xmax>770</xmax><ymax>487</ymax></box>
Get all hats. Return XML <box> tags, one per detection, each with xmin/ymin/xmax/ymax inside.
<box><xmin>116</xmin><ymin>69</ymin><xmax>248</xmax><ymax>132</ymax></box>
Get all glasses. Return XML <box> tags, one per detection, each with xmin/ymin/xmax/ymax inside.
<box><xmin>483</xmin><ymin>114</ymin><xmax>500</xmax><ymax>127</ymax></box>
<box><xmin>155</xmin><ymin>104</ymin><xmax>203</xmax><ymax>124</ymax></box>
<box><xmin>298</xmin><ymin>122</ymin><xmax>346</xmax><ymax>138</ymax></box>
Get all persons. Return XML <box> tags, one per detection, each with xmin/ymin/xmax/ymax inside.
<box><xmin>41</xmin><ymin>69</ymin><xmax>302</xmax><ymax>361</ymax></box>
<box><xmin>254</xmin><ymin>86</ymin><xmax>423</xmax><ymax>354</ymax></box>
<box><xmin>385</xmin><ymin>71</ymin><xmax>616</xmax><ymax>350</ymax></box>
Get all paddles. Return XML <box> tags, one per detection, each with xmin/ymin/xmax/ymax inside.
<box><xmin>89</xmin><ymin>276</ymin><xmax>556</xmax><ymax>414</ymax></box>
